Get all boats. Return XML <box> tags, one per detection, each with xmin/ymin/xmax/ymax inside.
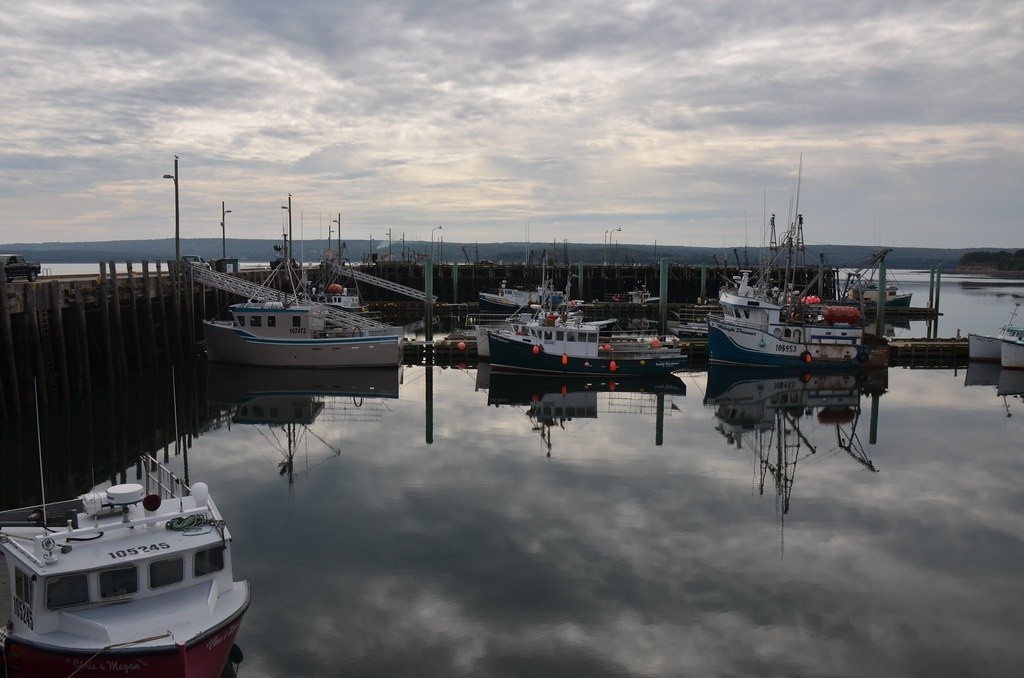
<box><xmin>202</xmin><ymin>214</ymin><xmax>404</xmax><ymax>369</ymax></box>
<box><xmin>1</xmin><ymin>366</ymin><xmax>250</xmax><ymax>678</ymax></box>
<box><xmin>963</xmin><ymin>362</ymin><xmax>1024</xmax><ymax>419</ymax></box>
<box><xmin>474</xmin><ymin>152</ymin><xmax>912</xmax><ymax>378</ymax></box>
<box><xmin>968</xmin><ymin>302</ymin><xmax>1024</xmax><ymax>370</ymax></box>
<box><xmin>191</xmin><ymin>352</ymin><xmax>404</xmax><ymax>503</ymax></box>
<box><xmin>703</xmin><ymin>365</ymin><xmax>880</xmax><ymax>561</ymax></box>
<box><xmin>475</xmin><ymin>360</ymin><xmax>687</xmax><ymax>458</ymax></box>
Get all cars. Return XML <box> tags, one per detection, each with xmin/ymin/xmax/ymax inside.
<box><xmin>480</xmin><ymin>260</ymin><xmax>494</xmax><ymax>267</ymax></box>
<box><xmin>656</xmin><ymin>258</ymin><xmax>680</xmax><ymax>267</ymax></box>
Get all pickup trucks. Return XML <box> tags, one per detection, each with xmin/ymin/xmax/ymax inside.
<box><xmin>172</xmin><ymin>255</ymin><xmax>210</xmax><ymax>275</ymax></box>
<box><xmin>0</xmin><ymin>254</ymin><xmax>41</xmax><ymax>282</ymax></box>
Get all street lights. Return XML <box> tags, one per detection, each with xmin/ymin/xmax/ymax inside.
<box><xmin>386</xmin><ymin>227</ymin><xmax>391</xmax><ymax>261</ymax></box>
<box><xmin>329</xmin><ymin>225</ymin><xmax>335</xmax><ymax>250</ymax></box>
<box><xmin>333</xmin><ymin>213</ymin><xmax>341</xmax><ymax>265</ymax></box>
<box><xmin>370</xmin><ymin>234</ymin><xmax>374</xmax><ymax>254</ymax></box>
<box><xmin>162</xmin><ymin>159</ymin><xmax>180</xmax><ymax>281</ymax></box>
<box><xmin>425</xmin><ymin>225</ymin><xmax>443</xmax><ymax>304</ymax></box>
<box><xmin>221</xmin><ymin>202</ymin><xmax>232</xmax><ymax>258</ymax></box>
<box><xmin>605</xmin><ymin>227</ymin><xmax>621</xmax><ymax>265</ymax></box>
<box><xmin>400</xmin><ymin>231</ymin><xmax>404</xmax><ymax>262</ymax></box>
<box><xmin>281</xmin><ymin>197</ymin><xmax>292</xmax><ymax>269</ymax></box>
